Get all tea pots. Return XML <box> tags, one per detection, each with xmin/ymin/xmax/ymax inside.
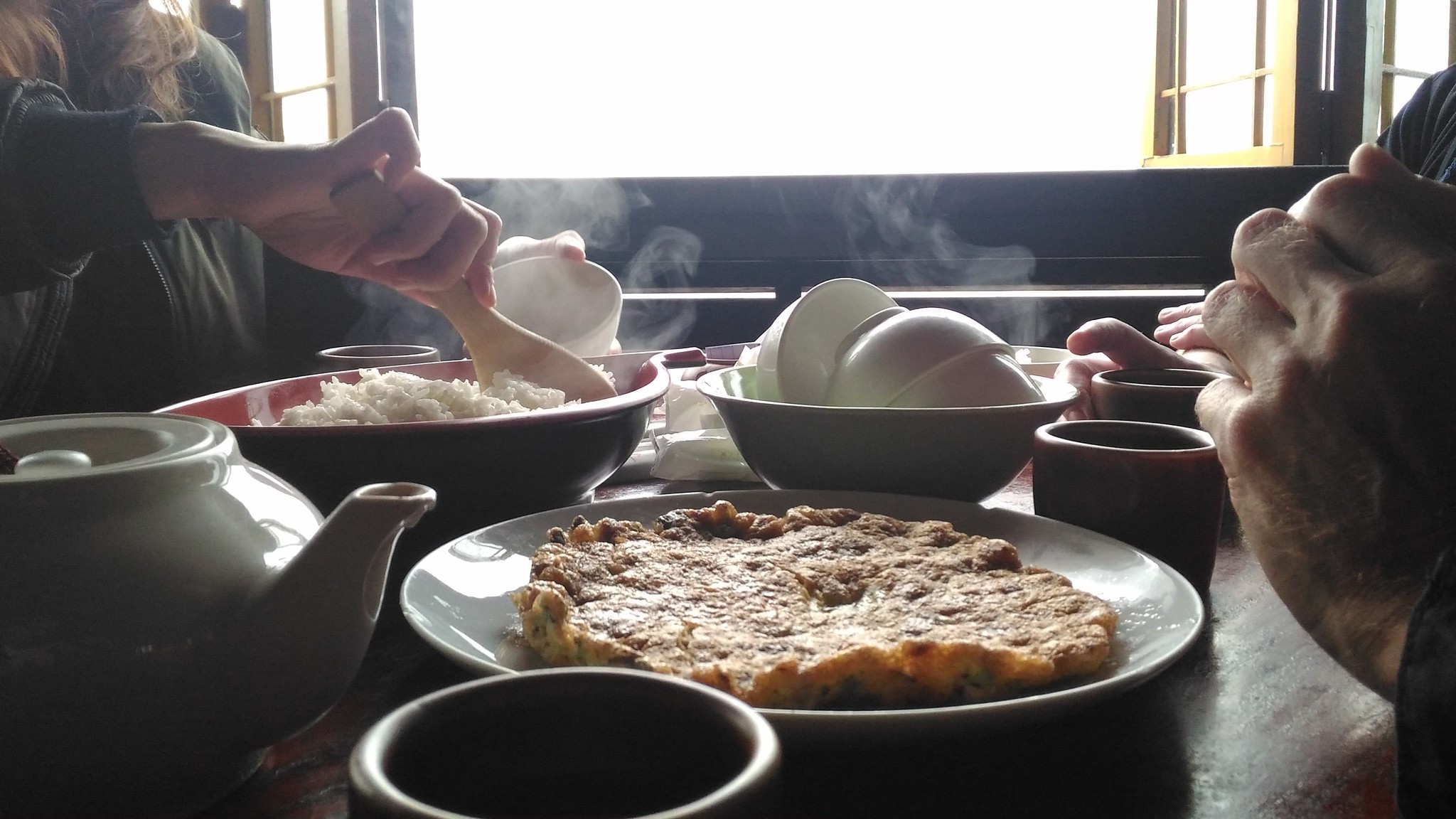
<box><xmin>1</xmin><ymin>410</ymin><xmax>437</xmax><ymax>819</ymax></box>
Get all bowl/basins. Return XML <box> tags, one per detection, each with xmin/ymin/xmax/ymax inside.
<box><xmin>827</xmin><ymin>306</ymin><xmax>1047</xmax><ymax>407</ymax></box>
<box><xmin>493</xmin><ymin>255</ymin><xmax>623</xmax><ymax>358</ymax></box>
<box><xmin>149</xmin><ymin>347</ymin><xmax>707</xmax><ymax>548</ymax></box>
<box><xmin>1012</xmin><ymin>345</ymin><xmax>1070</xmax><ymax>379</ymax></box>
<box><xmin>756</xmin><ymin>277</ymin><xmax>898</xmax><ymax>407</ymax></box>
<box><xmin>696</xmin><ymin>365</ymin><xmax>1082</xmax><ymax>505</ymax></box>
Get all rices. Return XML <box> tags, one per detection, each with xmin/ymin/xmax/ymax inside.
<box><xmin>249</xmin><ymin>360</ymin><xmax>616</xmax><ymax>425</ymax></box>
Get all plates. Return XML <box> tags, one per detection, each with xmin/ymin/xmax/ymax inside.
<box><xmin>399</xmin><ymin>490</ymin><xmax>1205</xmax><ymax>738</ymax></box>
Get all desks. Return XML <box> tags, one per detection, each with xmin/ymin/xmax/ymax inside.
<box><xmin>181</xmin><ymin>459</ymin><xmax>1400</xmax><ymax>819</ymax></box>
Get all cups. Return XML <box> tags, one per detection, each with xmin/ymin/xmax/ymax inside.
<box><xmin>1091</xmin><ymin>368</ymin><xmax>1233</xmax><ymax>431</ymax></box>
<box><xmin>1032</xmin><ymin>420</ymin><xmax>1227</xmax><ymax>593</ymax></box>
<box><xmin>309</xmin><ymin>345</ymin><xmax>440</xmax><ymax>375</ymax></box>
<box><xmin>349</xmin><ymin>666</ymin><xmax>783</xmax><ymax>819</ymax></box>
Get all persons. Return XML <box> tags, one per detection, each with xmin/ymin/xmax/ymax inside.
<box><xmin>0</xmin><ymin>0</ymin><xmax>502</xmax><ymax>410</ymax></box>
<box><xmin>1051</xmin><ymin>58</ymin><xmax>1456</xmax><ymax>819</ymax></box>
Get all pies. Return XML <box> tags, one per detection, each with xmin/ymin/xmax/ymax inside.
<box><xmin>518</xmin><ymin>496</ymin><xmax>1119</xmax><ymax>708</ymax></box>
